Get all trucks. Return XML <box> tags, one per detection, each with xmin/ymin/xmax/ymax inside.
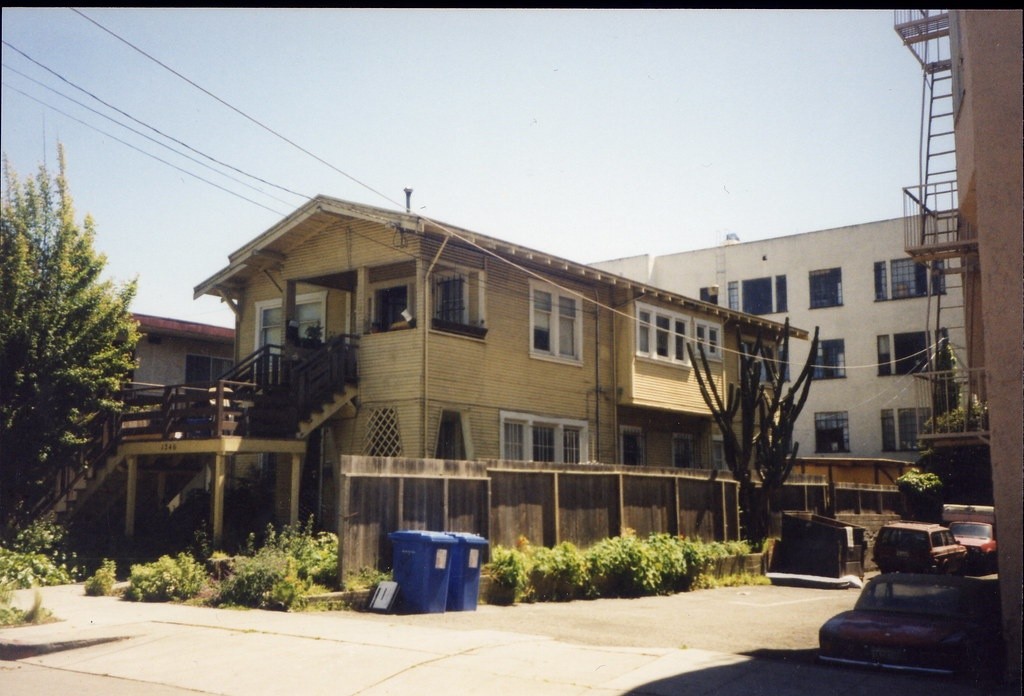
<box><xmin>941</xmin><ymin>505</ymin><xmax>997</xmax><ymax>570</ymax></box>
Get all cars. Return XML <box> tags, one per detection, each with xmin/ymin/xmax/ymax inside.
<box><xmin>816</xmin><ymin>572</ymin><xmax>1004</xmax><ymax>678</ymax></box>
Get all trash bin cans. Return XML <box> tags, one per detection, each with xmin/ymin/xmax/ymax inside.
<box><xmin>445</xmin><ymin>532</ymin><xmax>489</xmax><ymax>612</ymax></box>
<box><xmin>782</xmin><ymin>510</ymin><xmax>867</xmax><ymax>582</ymax></box>
<box><xmin>388</xmin><ymin>530</ymin><xmax>459</xmax><ymax>614</ymax></box>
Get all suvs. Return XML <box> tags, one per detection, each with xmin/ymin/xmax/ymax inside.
<box><xmin>872</xmin><ymin>522</ymin><xmax>969</xmax><ymax>578</ymax></box>
